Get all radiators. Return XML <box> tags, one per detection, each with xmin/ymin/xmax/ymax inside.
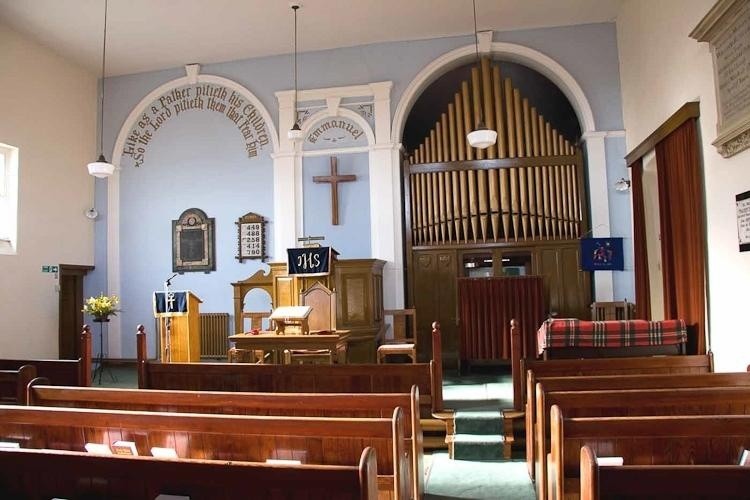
<box><xmin>200</xmin><ymin>313</ymin><xmax>228</xmax><ymax>360</ymax></box>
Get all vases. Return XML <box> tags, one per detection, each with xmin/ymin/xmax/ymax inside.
<box><xmin>94</xmin><ymin>311</ymin><xmax>108</xmax><ymax>321</ymax></box>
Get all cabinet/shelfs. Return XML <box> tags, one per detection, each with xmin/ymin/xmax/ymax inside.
<box><xmin>266</xmin><ymin>258</ymin><xmax>388</xmax><ymax>365</ymax></box>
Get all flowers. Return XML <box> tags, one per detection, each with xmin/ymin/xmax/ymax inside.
<box><xmin>80</xmin><ymin>291</ymin><xmax>119</xmax><ymax>314</ymax></box>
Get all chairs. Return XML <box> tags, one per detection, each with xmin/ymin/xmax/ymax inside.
<box><xmin>375</xmin><ymin>305</ymin><xmax>418</xmax><ymax>364</ymax></box>
<box><xmin>228</xmin><ymin>309</ymin><xmax>273</xmax><ymax>364</ymax></box>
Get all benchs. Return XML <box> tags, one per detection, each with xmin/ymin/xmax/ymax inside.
<box><xmin>517</xmin><ymin>348</ymin><xmax>750</xmax><ymax>500</ymax></box>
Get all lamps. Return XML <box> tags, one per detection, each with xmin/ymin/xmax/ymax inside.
<box><xmin>286</xmin><ymin>0</ymin><xmax>307</xmax><ymax>140</ymax></box>
<box><xmin>86</xmin><ymin>0</ymin><xmax>114</xmax><ymax>180</ymax></box>
<box><xmin>465</xmin><ymin>1</ymin><xmax>498</xmax><ymax>150</ymax></box>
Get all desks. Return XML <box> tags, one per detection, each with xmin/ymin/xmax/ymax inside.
<box><xmin>228</xmin><ymin>329</ymin><xmax>352</xmax><ymax>365</ymax></box>
